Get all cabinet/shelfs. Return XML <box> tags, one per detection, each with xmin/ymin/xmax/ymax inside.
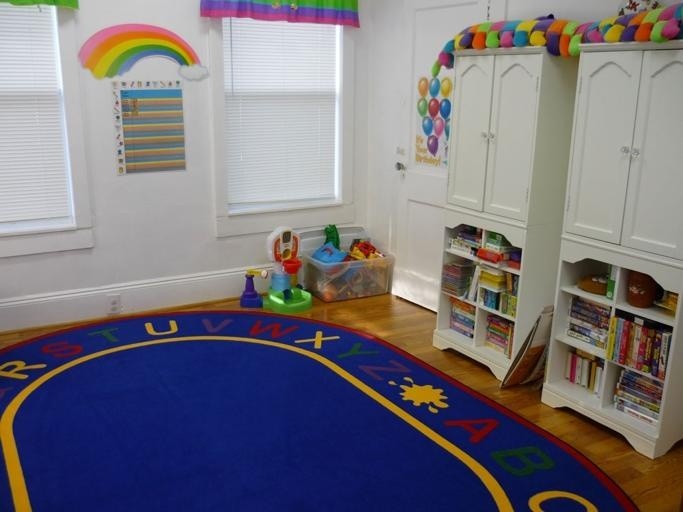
<box><xmin>431</xmin><ymin>209</ymin><xmax>561</xmax><ymax>381</ymax></box>
<box><xmin>541</xmin><ymin>239</ymin><xmax>682</xmax><ymax>460</ymax></box>
<box><xmin>442</xmin><ymin>46</ymin><xmax>577</xmax><ymax>224</ymax></box>
<box><xmin>561</xmin><ymin>37</ymin><xmax>682</xmax><ymax>264</ymax></box>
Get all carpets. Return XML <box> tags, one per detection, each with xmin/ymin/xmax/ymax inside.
<box><xmin>1</xmin><ymin>308</ymin><xmax>641</xmax><ymax>512</ymax></box>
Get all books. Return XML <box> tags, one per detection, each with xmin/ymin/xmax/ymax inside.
<box><xmin>442</xmin><ymin>230</ymin><xmax>522</xmax><ymax>359</ymax></box>
<box><xmin>565</xmin><ymin>300</ymin><xmax>673</xmax><ymax>426</ymax></box>
<box><xmin>654</xmin><ymin>293</ymin><xmax>679</xmax><ymax>312</ymax></box>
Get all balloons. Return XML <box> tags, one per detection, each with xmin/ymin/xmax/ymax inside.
<box><xmin>415</xmin><ymin>75</ymin><xmax>453</xmax><ymax>158</ymax></box>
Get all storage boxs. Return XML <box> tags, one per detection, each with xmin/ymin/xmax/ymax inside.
<box><xmin>300</xmin><ymin>247</ymin><xmax>395</xmax><ymax>303</ymax></box>
